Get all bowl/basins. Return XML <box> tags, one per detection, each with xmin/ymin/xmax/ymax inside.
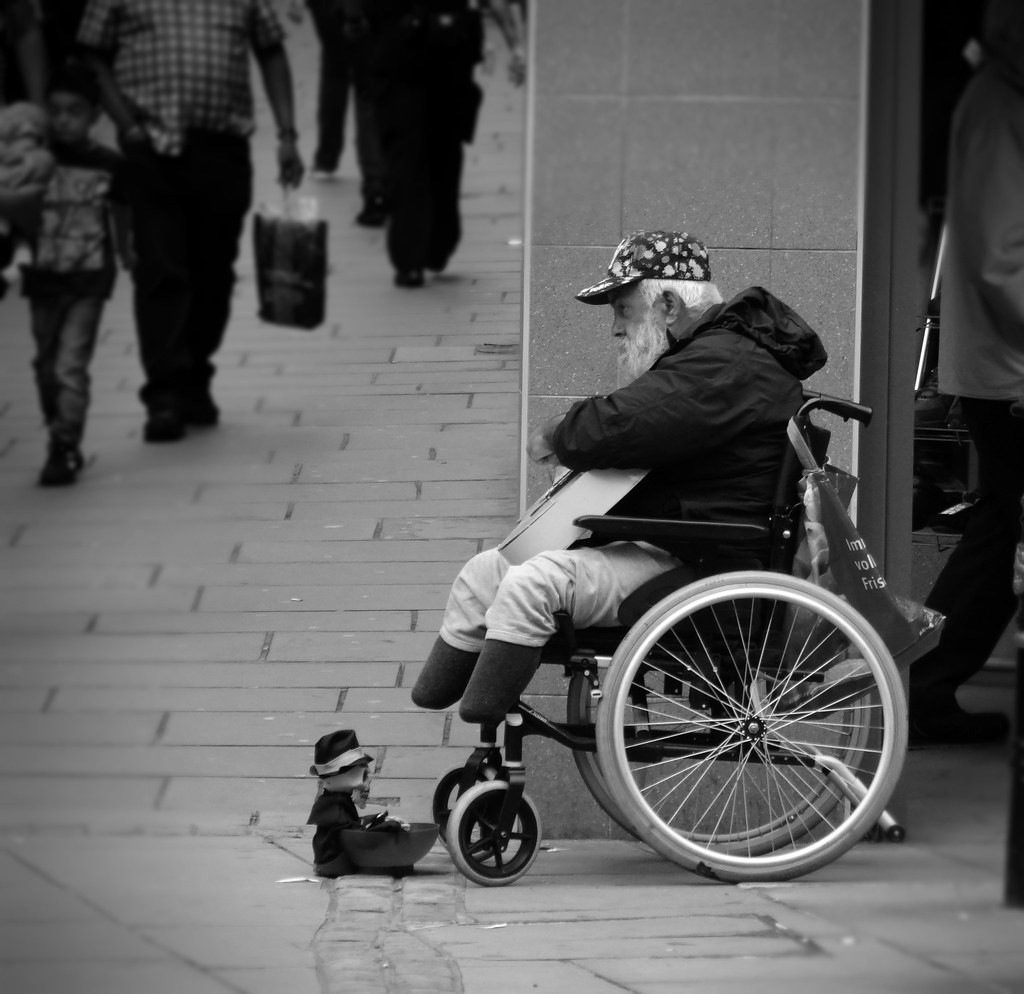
<box><xmin>340</xmin><ymin>821</ymin><xmax>440</xmax><ymax>868</ymax></box>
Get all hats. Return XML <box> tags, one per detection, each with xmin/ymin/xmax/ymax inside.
<box><xmin>575</xmin><ymin>228</ymin><xmax>711</xmax><ymax>305</ymax></box>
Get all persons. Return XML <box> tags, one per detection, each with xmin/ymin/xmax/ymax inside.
<box><xmin>409</xmin><ymin>219</ymin><xmax>830</xmax><ymax>722</ymax></box>
<box><xmin>72</xmin><ymin>1</ymin><xmax>318</xmax><ymax>440</ymax></box>
<box><xmin>0</xmin><ymin>1</ymin><xmax>119</xmax><ymax>489</ymax></box>
<box><xmin>293</xmin><ymin>0</ymin><xmax>527</xmax><ymax>292</ymax></box>
<box><xmin>898</xmin><ymin>3</ymin><xmax>1024</xmax><ymax>752</ymax></box>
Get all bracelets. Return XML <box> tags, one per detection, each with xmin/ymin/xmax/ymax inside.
<box><xmin>277</xmin><ymin>128</ymin><xmax>297</xmax><ymax>142</ymax></box>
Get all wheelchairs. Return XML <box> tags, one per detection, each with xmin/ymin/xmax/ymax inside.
<box><xmin>432</xmin><ymin>392</ymin><xmax>940</xmax><ymax>889</ymax></box>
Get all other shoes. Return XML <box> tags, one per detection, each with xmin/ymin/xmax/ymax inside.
<box><xmin>393</xmin><ymin>266</ymin><xmax>424</xmax><ymax>288</ymax></box>
<box><xmin>907</xmin><ymin>693</ymin><xmax>1009</xmax><ymax>751</ymax></box>
<box><xmin>141</xmin><ymin>380</ymin><xmax>222</xmax><ymax>446</ymax></box>
<box><xmin>34</xmin><ymin>436</ymin><xmax>88</xmax><ymax>486</ymax></box>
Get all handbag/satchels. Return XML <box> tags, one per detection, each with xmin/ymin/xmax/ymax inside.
<box><xmin>787</xmin><ymin>420</ymin><xmax>950</xmax><ymax>684</ymax></box>
<box><xmin>250</xmin><ymin>176</ymin><xmax>330</xmax><ymax>329</ymax></box>
